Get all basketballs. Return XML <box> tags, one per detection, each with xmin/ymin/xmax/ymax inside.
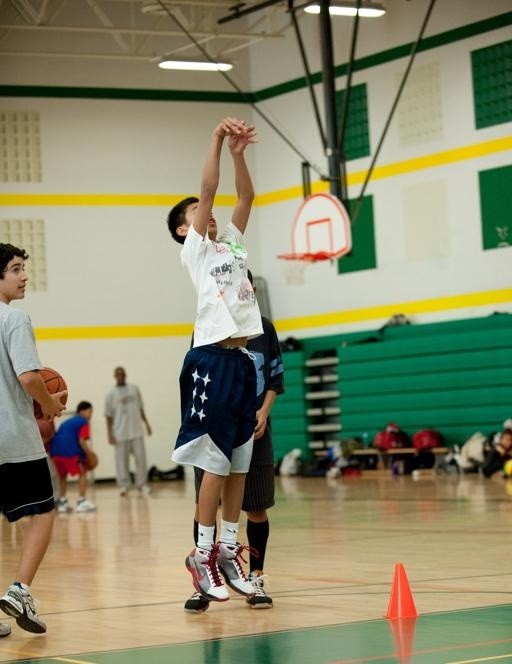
<box><xmin>33</xmin><ymin>368</ymin><xmax>68</xmax><ymax>420</ymax></box>
<box><xmin>84</xmin><ymin>451</ymin><xmax>98</xmax><ymax>470</ymax></box>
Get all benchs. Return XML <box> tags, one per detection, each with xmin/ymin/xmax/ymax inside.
<box><xmin>314</xmin><ymin>446</ymin><xmax>451</xmax><ymax>475</ymax></box>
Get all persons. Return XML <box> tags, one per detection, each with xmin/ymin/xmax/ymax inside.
<box><xmin>0</xmin><ymin>239</ymin><xmax>68</xmax><ymax>646</ymax></box>
<box><xmin>158</xmin><ymin>112</ymin><xmax>261</xmax><ymax>608</ymax></box>
<box><xmin>481</xmin><ymin>427</ymin><xmax>512</xmax><ymax>481</ymax></box>
<box><xmin>104</xmin><ymin>364</ymin><xmax>158</xmax><ymax>498</ymax></box>
<box><xmin>47</xmin><ymin>400</ymin><xmax>98</xmax><ymax>514</ymax></box>
<box><xmin>182</xmin><ymin>269</ymin><xmax>278</xmax><ymax>617</ymax></box>
<box><xmin>35</xmin><ymin>411</ymin><xmax>61</xmax><ymax>509</ymax></box>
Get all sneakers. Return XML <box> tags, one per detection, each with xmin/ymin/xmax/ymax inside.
<box><xmin>0</xmin><ymin>582</ymin><xmax>46</xmax><ymax>633</ymax></box>
<box><xmin>183</xmin><ymin>591</ymin><xmax>210</xmax><ymax>616</ymax></box>
<box><xmin>184</xmin><ymin>542</ymin><xmax>231</xmax><ymax>603</ymax></box>
<box><xmin>246</xmin><ymin>571</ymin><xmax>274</xmax><ymax>609</ymax></box>
<box><xmin>217</xmin><ymin>542</ymin><xmax>259</xmax><ymax>597</ymax></box>
<box><xmin>58</xmin><ymin>498</ymin><xmax>73</xmax><ymax>512</ymax></box>
<box><xmin>76</xmin><ymin>498</ymin><xmax>97</xmax><ymax>513</ymax></box>
<box><xmin>0</xmin><ymin>622</ymin><xmax>12</xmax><ymax>639</ymax></box>
